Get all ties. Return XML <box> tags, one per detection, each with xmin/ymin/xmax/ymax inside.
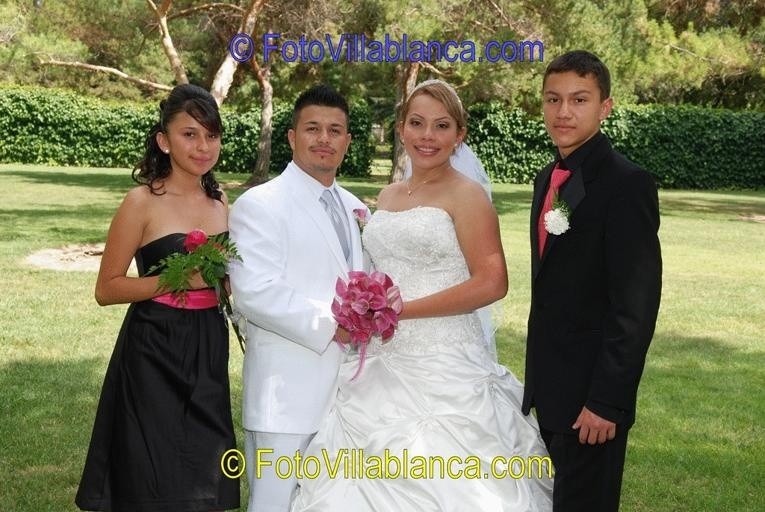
<box><xmin>537</xmin><ymin>162</ymin><xmax>572</xmax><ymax>260</ymax></box>
<box><xmin>318</xmin><ymin>190</ymin><xmax>350</xmax><ymax>263</ymax></box>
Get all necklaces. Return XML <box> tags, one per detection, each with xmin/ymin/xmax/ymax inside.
<box><xmin>403</xmin><ymin>173</ymin><xmax>438</xmax><ymax>197</ymax></box>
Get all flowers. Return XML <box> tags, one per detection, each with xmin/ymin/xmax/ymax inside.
<box><xmin>144</xmin><ymin>227</ymin><xmax>251</xmax><ymax>355</ymax></box>
<box><xmin>332</xmin><ymin>270</ymin><xmax>403</xmax><ymax>382</ymax></box>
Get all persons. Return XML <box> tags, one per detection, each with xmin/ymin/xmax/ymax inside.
<box><xmin>73</xmin><ymin>80</ymin><xmax>241</xmax><ymax>511</ymax></box>
<box><xmin>522</xmin><ymin>47</ymin><xmax>664</xmax><ymax>511</ymax></box>
<box><xmin>291</xmin><ymin>78</ymin><xmax>554</xmax><ymax>511</ymax></box>
<box><xmin>225</xmin><ymin>86</ymin><xmax>379</xmax><ymax>512</ymax></box>
